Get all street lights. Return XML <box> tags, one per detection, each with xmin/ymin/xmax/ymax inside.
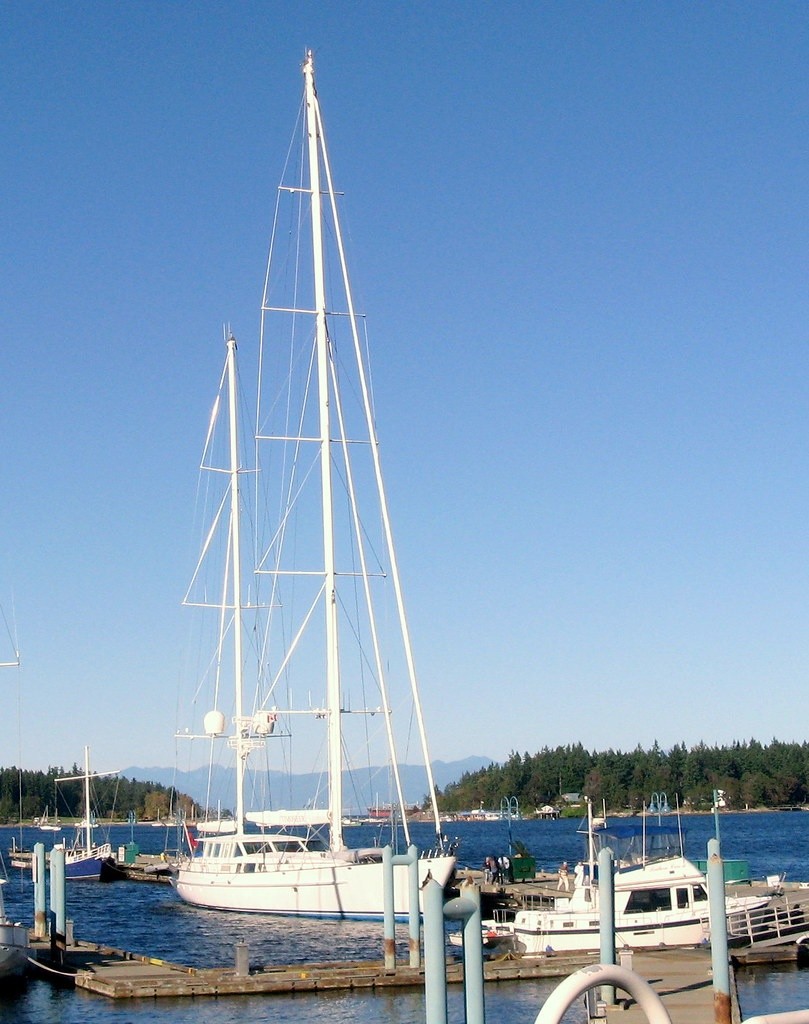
<box><xmin>648</xmin><ymin>791</ymin><xmax>670</xmax><ymax>846</ymax></box>
<box><xmin>498</xmin><ymin>795</ymin><xmax>520</xmax><ymax>856</ymax></box>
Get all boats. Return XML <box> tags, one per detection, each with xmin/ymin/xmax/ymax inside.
<box><xmin>353</xmin><ymin>817</ymin><xmax>387</xmax><ymax>823</ymax></box>
<box><xmin>591</xmin><ymin>816</ymin><xmax>606</xmax><ymax>831</ymax></box>
<box><xmin>341</xmin><ymin>817</ymin><xmax>360</xmax><ymax>826</ymax></box>
<box><xmin>100</xmin><ymin>754</ymin><xmax>183</xmax><ymax>886</ymax></box>
<box><xmin>450</xmin><ymin>791</ymin><xmax>771</xmax><ymax>957</ymax></box>
<box><xmin>74</xmin><ymin>818</ymin><xmax>98</xmax><ymax>829</ymax></box>
<box><xmin>367</xmin><ymin>803</ymin><xmax>417</xmax><ymax>817</ymax></box>
<box><xmin>32</xmin><ymin>804</ymin><xmax>61</xmax><ymax>831</ymax></box>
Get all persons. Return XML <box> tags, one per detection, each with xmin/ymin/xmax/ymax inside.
<box><xmin>556</xmin><ymin>862</ymin><xmax>570</xmax><ymax>892</ymax></box>
<box><xmin>574</xmin><ymin>861</ymin><xmax>585</xmax><ymax>885</ymax></box>
<box><xmin>485</xmin><ymin>854</ymin><xmax>515</xmax><ymax>885</ymax></box>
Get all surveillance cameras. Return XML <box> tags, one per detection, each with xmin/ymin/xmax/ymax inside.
<box><xmin>719</xmin><ymin>800</ymin><xmax>725</xmax><ymax>808</ymax></box>
<box><xmin>718</xmin><ymin>789</ymin><xmax>726</xmax><ymax>795</ymax></box>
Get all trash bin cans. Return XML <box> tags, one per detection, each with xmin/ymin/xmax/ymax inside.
<box><xmin>504</xmin><ymin>857</ymin><xmax>535</xmax><ymax>879</ymax></box>
<box><xmin>117</xmin><ymin>844</ymin><xmax>139</xmax><ymax>863</ymax></box>
<box><xmin>690</xmin><ymin>859</ymin><xmax>749</xmax><ymax>883</ymax></box>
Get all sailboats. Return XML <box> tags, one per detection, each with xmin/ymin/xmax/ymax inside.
<box><xmin>51</xmin><ymin>746</ymin><xmax>120</xmax><ymax>882</ymax></box>
<box><xmin>178</xmin><ymin>33</ymin><xmax>458</xmax><ymax>921</ymax></box>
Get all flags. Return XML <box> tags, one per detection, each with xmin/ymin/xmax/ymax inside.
<box><xmin>187</xmin><ymin>827</ymin><xmax>198</xmax><ymax>847</ymax></box>
